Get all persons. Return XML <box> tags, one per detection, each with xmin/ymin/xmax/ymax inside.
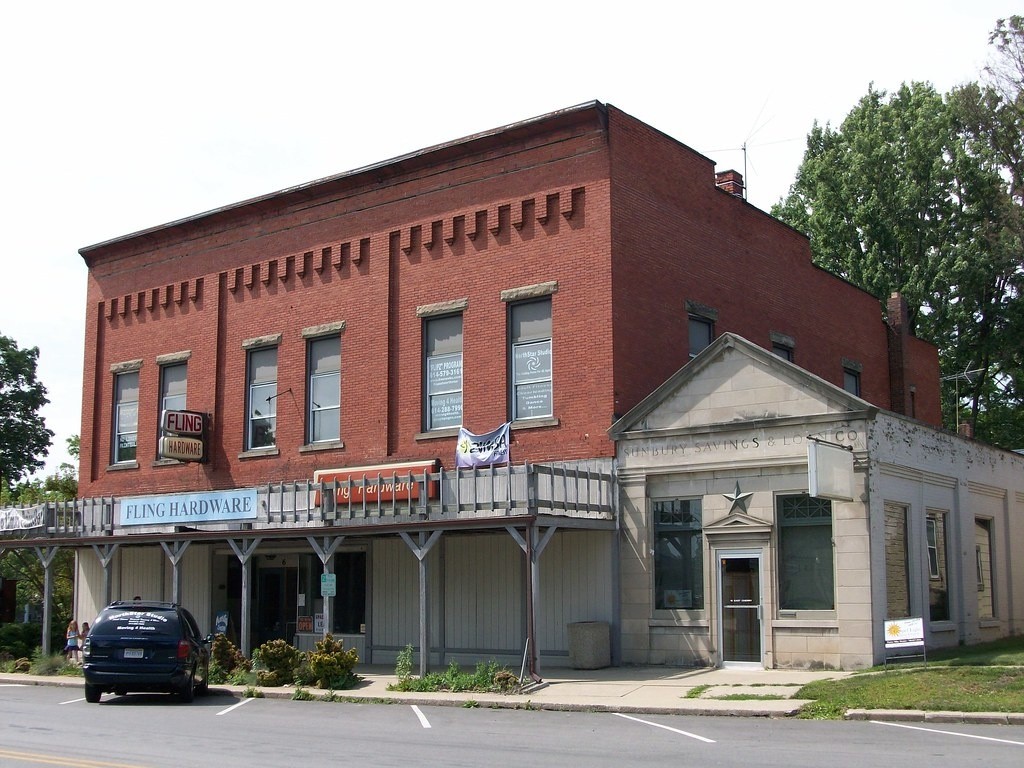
<box><xmin>64</xmin><ymin>621</ymin><xmax>90</xmax><ymax>660</ymax></box>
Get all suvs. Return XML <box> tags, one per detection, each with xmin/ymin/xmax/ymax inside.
<box><xmin>82</xmin><ymin>600</ymin><xmax>215</xmax><ymax>703</ymax></box>
